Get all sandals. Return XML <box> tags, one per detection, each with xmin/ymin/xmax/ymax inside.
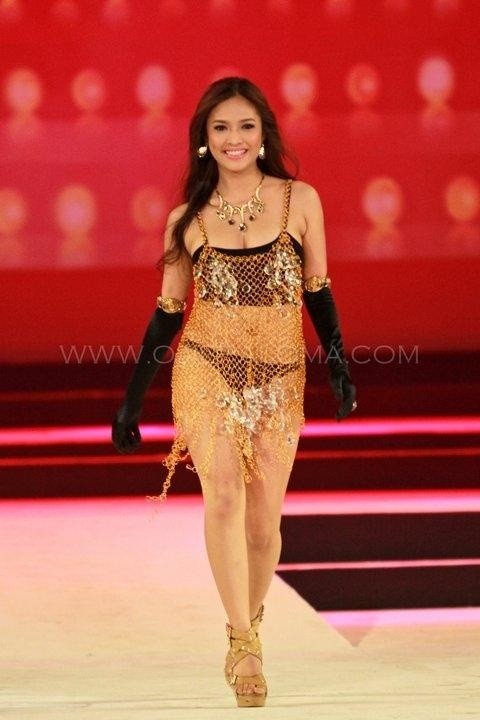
<box><xmin>224</xmin><ymin>607</ymin><xmax>268</xmax><ymax>708</ymax></box>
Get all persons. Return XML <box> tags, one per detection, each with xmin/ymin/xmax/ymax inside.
<box><xmin>110</xmin><ymin>75</ymin><xmax>358</xmax><ymax>706</ymax></box>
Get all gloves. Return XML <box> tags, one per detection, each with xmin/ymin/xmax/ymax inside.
<box><xmin>111</xmin><ymin>308</ymin><xmax>186</xmax><ymax>451</ymax></box>
<box><xmin>302</xmin><ymin>288</ymin><xmax>356</xmax><ymax>419</ymax></box>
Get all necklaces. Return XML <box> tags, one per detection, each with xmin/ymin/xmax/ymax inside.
<box><xmin>212</xmin><ymin>172</ymin><xmax>266</xmax><ymax>231</ymax></box>
